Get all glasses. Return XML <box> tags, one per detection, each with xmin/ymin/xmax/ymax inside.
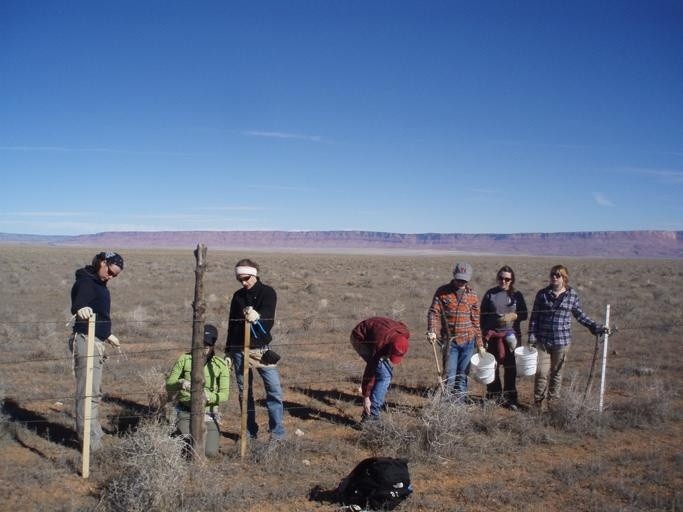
<box><xmin>499</xmin><ymin>277</ymin><xmax>512</xmax><ymax>282</ymax></box>
<box><xmin>107</xmin><ymin>264</ymin><xmax>117</xmax><ymax>277</ymax></box>
<box><xmin>238</xmin><ymin>275</ymin><xmax>251</xmax><ymax>282</ymax></box>
<box><xmin>551</xmin><ymin>271</ymin><xmax>562</xmax><ymax>278</ymax></box>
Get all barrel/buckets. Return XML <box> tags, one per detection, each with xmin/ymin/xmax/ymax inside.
<box><xmin>471</xmin><ymin>353</ymin><xmax>497</xmax><ymax>385</ymax></box>
<box><xmin>514</xmin><ymin>345</ymin><xmax>538</xmax><ymax>376</ymax></box>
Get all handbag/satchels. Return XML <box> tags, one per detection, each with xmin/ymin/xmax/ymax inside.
<box><xmin>336</xmin><ymin>457</ymin><xmax>412</xmax><ymax>512</ymax></box>
<box><xmin>261</xmin><ymin>350</ymin><xmax>280</xmax><ymax>364</ymax></box>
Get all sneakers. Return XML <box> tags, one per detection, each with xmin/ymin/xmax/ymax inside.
<box><xmin>361</xmin><ymin>412</ymin><xmax>379</xmax><ymax>421</ymax></box>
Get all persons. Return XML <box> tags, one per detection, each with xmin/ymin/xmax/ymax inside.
<box><xmin>68</xmin><ymin>251</ymin><xmax>124</xmax><ymax>453</ymax></box>
<box><xmin>527</xmin><ymin>265</ymin><xmax>612</xmax><ymax>413</ymax></box>
<box><xmin>224</xmin><ymin>259</ymin><xmax>286</xmax><ymax>452</ymax></box>
<box><xmin>426</xmin><ymin>263</ymin><xmax>480</xmax><ymax>402</ymax></box>
<box><xmin>166</xmin><ymin>325</ymin><xmax>230</xmax><ymax>459</ymax></box>
<box><xmin>480</xmin><ymin>265</ymin><xmax>528</xmax><ymax>411</ymax></box>
<box><xmin>350</xmin><ymin>317</ymin><xmax>410</xmax><ymax>425</ymax></box>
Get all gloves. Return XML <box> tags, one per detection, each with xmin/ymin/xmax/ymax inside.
<box><xmin>107</xmin><ymin>335</ymin><xmax>120</xmax><ymax>346</ymax></box>
<box><xmin>178</xmin><ymin>379</ymin><xmax>191</xmax><ymax>389</ymax></box>
<box><xmin>243</xmin><ymin>306</ymin><xmax>260</xmax><ymax>321</ymax></box>
<box><xmin>478</xmin><ymin>346</ymin><xmax>486</xmax><ymax>358</ymax></box>
<box><xmin>427</xmin><ymin>332</ymin><xmax>436</xmax><ymax>344</ymax></box>
<box><xmin>78</xmin><ymin>306</ymin><xmax>94</xmax><ymax>319</ymax></box>
<box><xmin>204</xmin><ymin>388</ymin><xmax>211</xmax><ymax>402</ymax></box>
<box><xmin>498</xmin><ymin>313</ymin><xmax>517</xmax><ymax>325</ymax></box>
<box><xmin>225</xmin><ymin>356</ymin><xmax>234</xmax><ymax>369</ymax></box>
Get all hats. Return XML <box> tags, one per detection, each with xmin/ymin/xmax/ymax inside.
<box><xmin>455</xmin><ymin>264</ymin><xmax>472</xmax><ymax>281</ymax></box>
<box><xmin>203</xmin><ymin>325</ymin><xmax>218</xmax><ymax>345</ymax></box>
<box><xmin>390</xmin><ymin>336</ymin><xmax>408</xmax><ymax>363</ymax></box>
